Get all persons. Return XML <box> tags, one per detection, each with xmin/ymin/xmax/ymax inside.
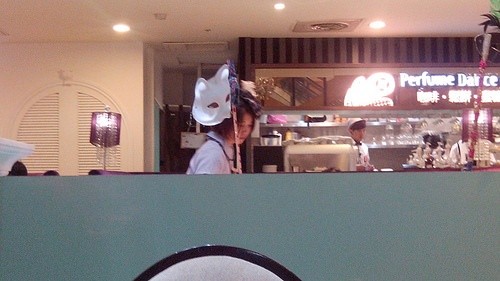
<box><xmin>450</xmin><ymin>133</ymin><xmax>478</xmax><ymax>168</ymax></box>
<box><xmin>186</xmin><ymin>103</ymin><xmax>256</xmax><ymax>175</ymax></box>
<box><xmin>43</xmin><ymin>170</ymin><xmax>61</xmax><ymax>177</ymax></box>
<box><xmin>7</xmin><ymin>161</ymin><xmax>28</xmax><ymax>177</ymax></box>
<box><xmin>89</xmin><ymin>170</ymin><xmax>102</xmax><ymax>175</ymax></box>
<box><xmin>347</xmin><ymin>117</ymin><xmax>376</xmax><ymax>171</ymax></box>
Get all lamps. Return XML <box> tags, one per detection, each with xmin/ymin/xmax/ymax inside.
<box><xmin>90</xmin><ymin>112</ymin><xmax>121</xmax><ymax>170</ymax></box>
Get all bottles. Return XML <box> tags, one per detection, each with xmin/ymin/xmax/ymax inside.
<box><xmin>285</xmin><ymin>129</ymin><xmax>292</xmax><ymax>140</ymax></box>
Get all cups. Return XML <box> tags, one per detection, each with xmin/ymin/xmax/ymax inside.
<box><xmin>379</xmin><ymin>136</ymin><xmax>421</xmax><ymax>144</ymax></box>
<box><xmin>293</xmin><ymin>165</ymin><xmax>299</xmax><ymax>173</ymax></box>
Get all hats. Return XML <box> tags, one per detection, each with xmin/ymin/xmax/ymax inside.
<box><xmin>347</xmin><ymin>117</ymin><xmax>365</xmax><ymax>131</ymax></box>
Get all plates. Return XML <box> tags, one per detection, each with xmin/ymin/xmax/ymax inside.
<box><xmin>261</xmin><ymin>165</ymin><xmax>277</xmax><ymax>173</ymax></box>
